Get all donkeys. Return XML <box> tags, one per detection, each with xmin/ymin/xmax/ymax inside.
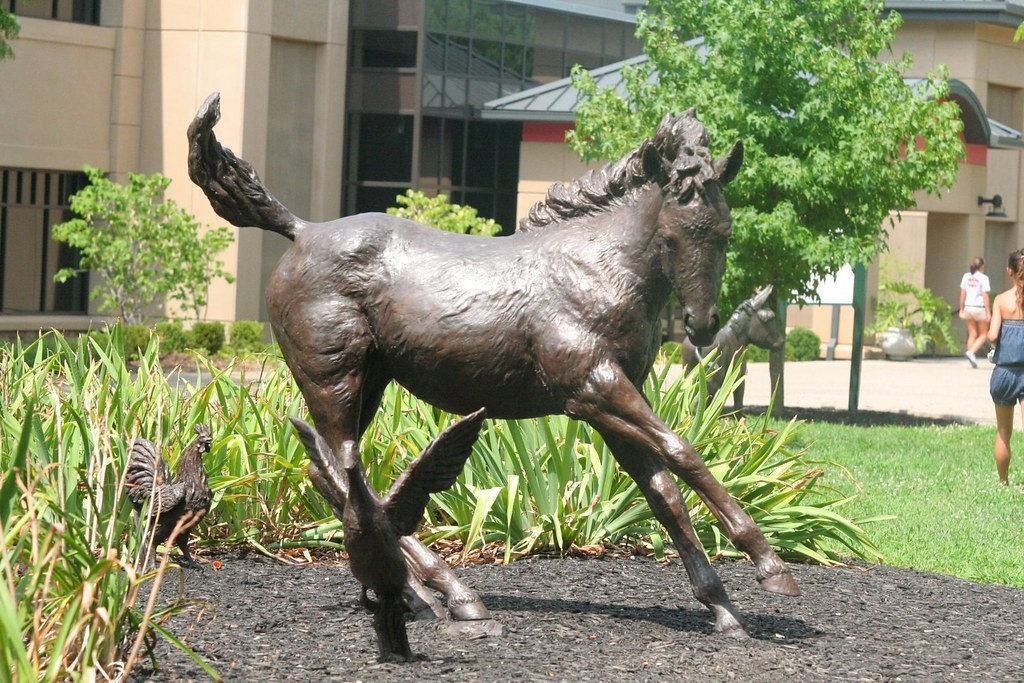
<box><xmin>682</xmin><ymin>283</ymin><xmax>785</xmax><ymax>422</ymax></box>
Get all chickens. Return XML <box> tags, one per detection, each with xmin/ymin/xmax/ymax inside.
<box><xmin>124</xmin><ymin>422</ymin><xmax>215</xmax><ymax>573</ymax></box>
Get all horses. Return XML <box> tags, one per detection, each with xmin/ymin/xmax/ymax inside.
<box><xmin>186</xmin><ymin>90</ymin><xmax>802</xmax><ymax>636</ymax></box>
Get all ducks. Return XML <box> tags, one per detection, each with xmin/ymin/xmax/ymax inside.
<box><xmin>287</xmin><ymin>407</ymin><xmax>490</xmax><ymax>623</ymax></box>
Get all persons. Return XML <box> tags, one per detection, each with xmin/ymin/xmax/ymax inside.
<box><xmin>986</xmin><ymin>248</ymin><xmax>1024</xmax><ymax>487</ymax></box>
<box><xmin>958</xmin><ymin>257</ymin><xmax>992</xmax><ymax>368</ymax></box>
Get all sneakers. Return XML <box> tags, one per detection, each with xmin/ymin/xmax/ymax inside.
<box><xmin>965</xmin><ymin>350</ymin><xmax>978</xmax><ymax>369</ymax></box>
